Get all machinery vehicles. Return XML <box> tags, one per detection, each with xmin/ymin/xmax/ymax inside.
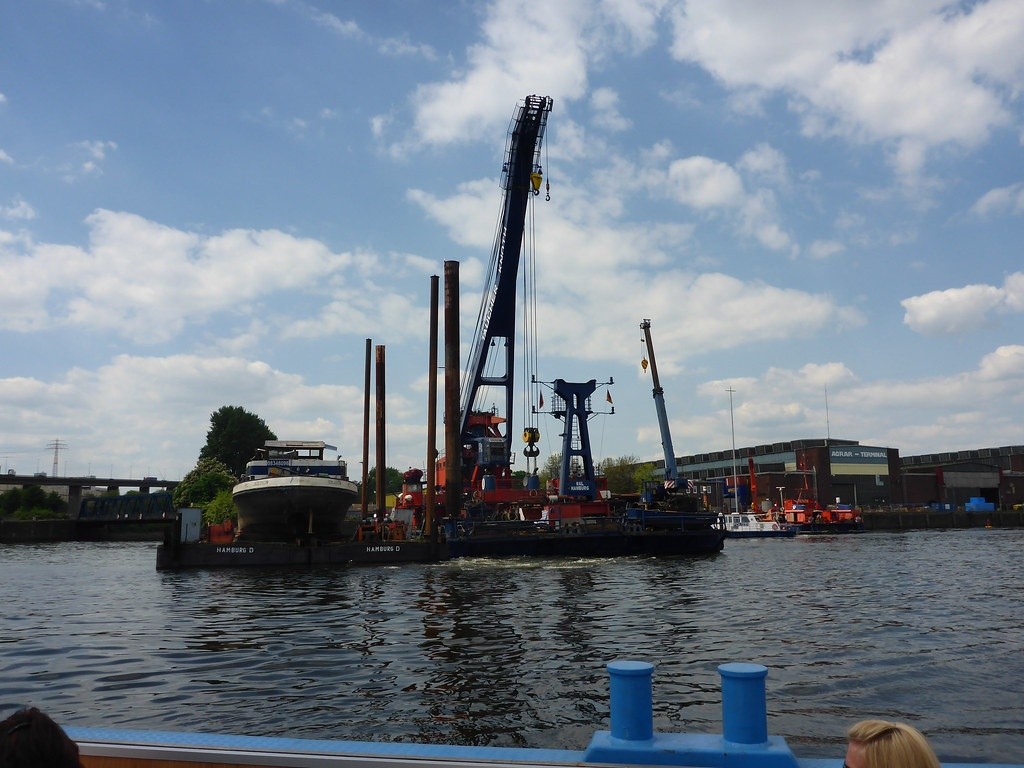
<box><xmin>400</xmin><ymin>86</ymin><xmax>566</xmax><ymax>524</ymax></box>
<box><xmin>622</xmin><ymin>313</ymin><xmax>721</xmax><ymax>527</ymax></box>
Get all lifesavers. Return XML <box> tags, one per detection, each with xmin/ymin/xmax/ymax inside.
<box><xmin>529</xmin><ymin>489</ymin><xmax>539</xmax><ymax>498</ymax></box>
<box><xmin>472</xmin><ymin>490</ymin><xmax>482</xmax><ymax>499</ymax></box>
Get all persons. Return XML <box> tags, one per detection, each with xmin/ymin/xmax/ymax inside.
<box><xmin>842</xmin><ymin>720</ymin><xmax>940</xmax><ymax>768</ymax></box>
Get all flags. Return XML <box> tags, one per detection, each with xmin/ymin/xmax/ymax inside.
<box><xmin>606</xmin><ymin>390</ymin><xmax>613</xmax><ymax>404</ymax></box>
<box><xmin>539</xmin><ymin>390</ymin><xmax>543</xmax><ymax>408</ymax></box>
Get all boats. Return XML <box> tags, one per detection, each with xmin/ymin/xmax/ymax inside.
<box><xmin>709</xmin><ymin>512</ymin><xmax>800</xmax><ymax>536</ymax></box>
<box><xmin>228</xmin><ymin>439</ymin><xmax>361</xmax><ymax>535</ymax></box>
<box><xmin>151</xmin><ymin>258</ymin><xmax>728</xmax><ymax>569</ymax></box>
<box><xmin>746</xmin><ymin>455</ymin><xmax>867</xmax><ymax>531</ymax></box>
